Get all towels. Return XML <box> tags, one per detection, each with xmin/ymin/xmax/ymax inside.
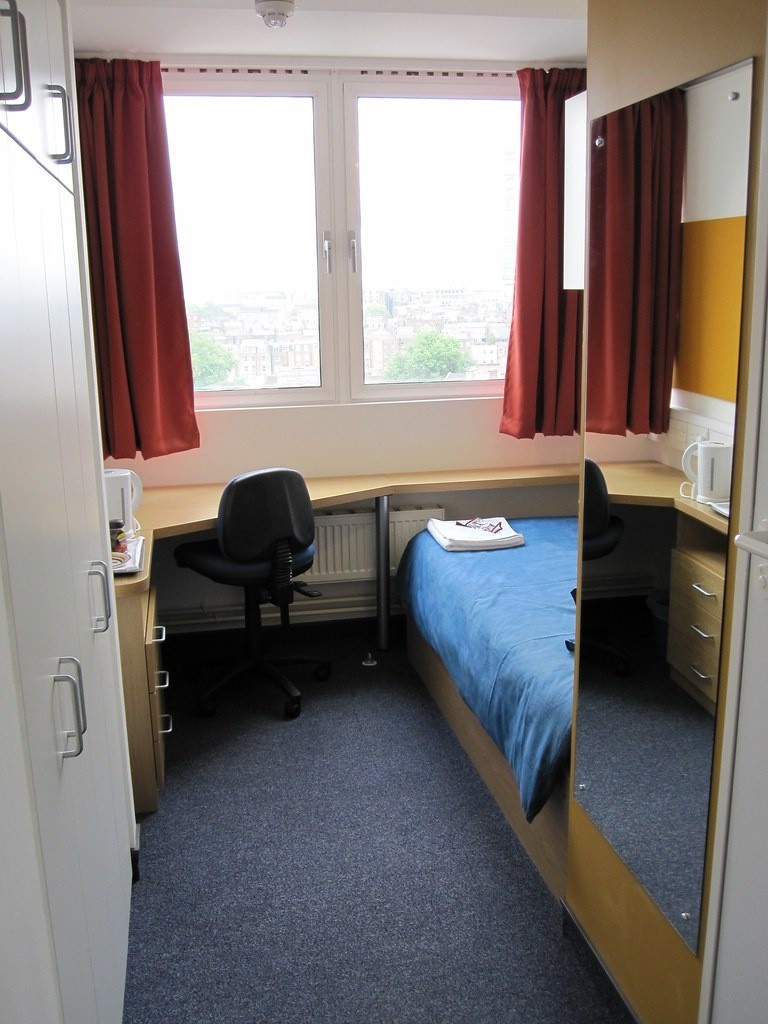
<box><xmin>427</xmin><ymin>516</ymin><xmax>525</xmax><ymax>553</ymax></box>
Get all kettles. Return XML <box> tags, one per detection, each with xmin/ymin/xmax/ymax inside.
<box><xmin>104</xmin><ymin>468</ymin><xmax>143</xmax><ymax>539</ymax></box>
<box><xmin>681</xmin><ymin>439</ymin><xmax>732</xmax><ymax>502</ymax></box>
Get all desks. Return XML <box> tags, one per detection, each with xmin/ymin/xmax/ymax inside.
<box><xmin>113</xmin><ymin>463</ymin><xmax>579</xmax><ymax>813</ymax></box>
<box><xmin>595</xmin><ymin>459</ymin><xmax>729</xmax><ymax>535</ymax></box>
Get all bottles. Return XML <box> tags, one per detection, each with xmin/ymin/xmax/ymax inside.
<box><xmin>110</xmin><ymin>519</ymin><xmax>127</xmax><ymax>554</ymax></box>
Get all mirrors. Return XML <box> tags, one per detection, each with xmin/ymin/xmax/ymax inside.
<box><xmin>570</xmin><ymin>57</ymin><xmax>755</xmax><ymax>957</ymax></box>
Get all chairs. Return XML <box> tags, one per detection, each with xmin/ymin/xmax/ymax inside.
<box><xmin>171</xmin><ymin>470</ymin><xmax>330</xmax><ymax>721</ymax></box>
<box><xmin>582</xmin><ymin>457</ymin><xmax>632</xmax><ymax>678</ymax></box>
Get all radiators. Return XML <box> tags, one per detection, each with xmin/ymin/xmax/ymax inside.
<box><xmin>290</xmin><ymin>503</ymin><xmax>445</xmax><ymax>585</ymax></box>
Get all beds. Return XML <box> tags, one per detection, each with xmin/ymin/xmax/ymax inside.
<box><xmin>391</xmin><ymin>517</ymin><xmax>580</xmax><ymax>907</ymax></box>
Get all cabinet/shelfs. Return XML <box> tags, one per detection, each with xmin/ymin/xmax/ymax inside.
<box><xmin>0</xmin><ymin>128</ymin><xmax>133</xmax><ymax>1024</ymax></box>
<box><xmin>115</xmin><ymin>585</ymin><xmax>172</xmax><ymax>813</ymax></box>
<box><xmin>680</xmin><ymin>62</ymin><xmax>752</xmax><ymax>223</ymax></box>
<box><xmin>666</xmin><ymin>511</ymin><xmax>728</xmax><ymax>716</ymax></box>
<box><xmin>0</xmin><ymin>0</ymin><xmax>78</xmax><ymax>195</ymax></box>
